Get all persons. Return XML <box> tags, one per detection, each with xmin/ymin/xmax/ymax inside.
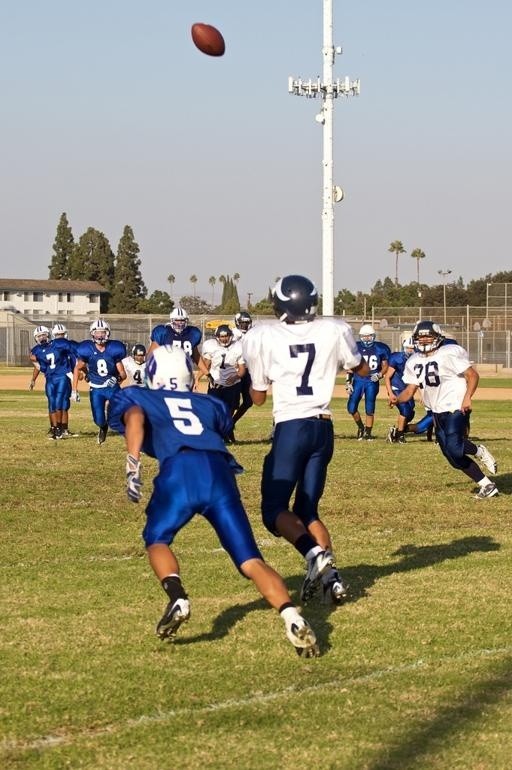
<box><xmin>389</xmin><ymin>321</ymin><xmax>497</xmax><ymax>499</ymax></box>
<box><xmin>72</xmin><ymin>320</ymin><xmax>126</xmax><ymax>442</ymax></box>
<box><xmin>29</xmin><ymin>325</ymin><xmax>84</xmax><ymax>436</ymax></box>
<box><xmin>26</xmin><ymin>325</ymin><xmax>90</xmax><ymax>439</ymax></box>
<box><xmin>384</xmin><ymin>337</ymin><xmax>424</xmax><ymax>442</ymax></box>
<box><xmin>104</xmin><ymin>383</ymin><xmax>321</xmax><ymax>662</ymax></box>
<box><xmin>239</xmin><ymin>276</ymin><xmax>372</xmax><ymax>606</ymax></box>
<box><xmin>345</xmin><ymin>324</ymin><xmax>391</xmax><ymax>440</ymax></box>
<box><xmin>389</xmin><ymin>323</ymin><xmax>497</xmax><ymax>499</ymax></box>
<box><xmin>140</xmin><ymin>324</ymin><xmax>192</xmax><ymax>392</ymax></box>
<box><xmin>147</xmin><ymin>311</ymin><xmax>202</xmax><ymax>365</ymax></box>
<box><xmin>116</xmin><ymin>344</ymin><xmax>146</xmax><ymax>389</ymax></box>
<box><xmin>224</xmin><ymin>311</ymin><xmax>253</xmax><ymax>442</ymax></box>
<box><xmin>198</xmin><ymin>324</ymin><xmax>245</xmax><ymax>416</ymax></box>
<box><xmin>389</xmin><ymin>415</ymin><xmax>433</xmax><ymax>442</ymax></box>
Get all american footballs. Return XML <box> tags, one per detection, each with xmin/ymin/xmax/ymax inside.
<box><xmin>192</xmin><ymin>23</ymin><xmax>225</xmax><ymax>57</ymax></box>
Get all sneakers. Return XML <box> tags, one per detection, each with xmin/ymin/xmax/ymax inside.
<box><xmin>45</xmin><ymin>423</ymin><xmax>80</xmax><ymax>441</ymax></box>
<box><xmin>478</xmin><ymin>444</ymin><xmax>497</xmax><ymax>474</ymax></box>
<box><xmin>472</xmin><ymin>483</ymin><xmax>499</xmax><ymax>500</ymax></box>
<box><xmin>287</xmin><ymin>620</ymin><xmax>321</xmax><ymax>659</ymax></box>
<box><xmin>364</xmin><ymin>432</ymin><xmax>373</xmax><ymax>441</ymax></box>
<box><xmin>300</xmin><ymin>548</ymin><xmax>337</xmax><ymax>603</ymax></box>
<box><xmin>357</xmin><ymin>428</ymin><xmax>364</xmax><ymax>441</ymax></box>
<box><xmin>321</xmin><ymin>570</ymin><xmax>347</xmax><ymax>605</ymax></box>
<box><xmin>385</xmin><ymin>426</ymin><xmax>408</xmax><ymax>444</ymax></box>
<box><xmin>97</xmin><ymin>423</ymin><xmax>109</xmax><ymax>445</ymax></box>
<box><xmin>156</xmin><ymin>597</ymin><xmax>192</xmax><ymax>642</ymax></box>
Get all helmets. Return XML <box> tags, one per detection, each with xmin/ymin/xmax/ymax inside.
<box><xmin>214</xmin><ymin>323</ymin><xmax>233</xmax><ymax>347</ymax></box>
<box><xmin>234</xmin><ymin>311</ymin><xmax>252</xmax><ymax>332</ymax></box>
<box><xmin>359</xmin><ymin>324</ymin><xmax>376</xmax><ymax>346</ymax></box>
<box><xmin>33</xmin><ymin>326</ymin><xmax>50</xmax><ymax>345</ymax></box>
<box><xmin>412</xmin><ymin>319</ymin><xmax>444</xmax><ymax>354</ymax></box>
<box><xmin>90</xmin><ymin>320</ymin><xmax>111</xmax><ymax>343</ymax></box>
<box><xmin>132</xmin><ymin>344</ymin><xmax>146</xmax><ymax>364</ymax></box>
<box><xmin>170</xmin><ymin>307</ymin><xmax>189</xmax><ymax>333</ymax></box>
<box><xmin>52</xmin><ymin>323</ymin><xmax>67</xmax><ymax>337</ymax></box>
<box><xmin>402</xmin><ymin>334</ymin><xmax>412</xmax><ymax>352</ymax></box>
<box><xmin>271</xmin><ymin>274</ymin><xmax>318</xmax><ymax>324</ymax></box>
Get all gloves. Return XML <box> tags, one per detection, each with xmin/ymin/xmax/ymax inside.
<box><xmin>123</xmin><ymin>451</ymin><xmax>145</xmax><ymax>504</ymax></box>
<box><xmin>345</xmin><ymin>381</ymin><xmax>354</xmax><ymax>394</ymax></box>
<box><xmin>84</xmin><ymin>373</ymin><xmax>91</xmax><ymax>383</ymax></box>
<box><xmin>106</xmin><ymin>375</ymin><xmax>122</xmax><ymax>388</ymax></box>
<box><xmin>70</xmin><ymin>390</ymin><xmax>80</xmax><ymax>403</ymax></box>
<box><xmin>28</xmin><ymin>379</ymin><xmax>36</xmax><ymax>392</ymax></box>
<box><xmin>370</xmin><ymin>372</ymin><xmax>382</xmax><ymax>383</ymax></box>
